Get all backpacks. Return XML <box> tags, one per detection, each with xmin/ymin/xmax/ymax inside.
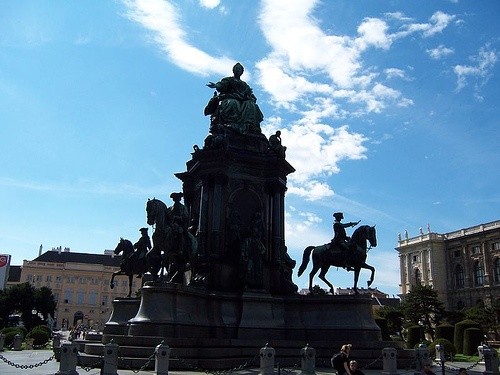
<box><xmin>331</xmin><ymin>354</ymin><xmax>344</xmax><ymax>370</ymax></box>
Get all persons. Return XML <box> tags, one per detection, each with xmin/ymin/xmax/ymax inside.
<box><xmin>162</xmin><ymin>192</ymin><xmax>190</xmax><ymax>257</ymax></box>
<box><xmin>423</xmin><ymin>363</ymin><xmax>436</xmax><ymax>375</ymax></box>
<box><xmin>333</xmin><ymin>344</ymin><xmax>353</xmax><ymax>375</ymax></box>
<box><xmin>458</xmin><ymin>367</ymin><xmax>470</xmax><ymax>375</ymax></box>
<box><xmin>240</xmin><ymin>226</ymin><xmax>268</xmax><ymax>293</ymax></box>
<box><xmin>205</xmin><ymin>63</ymin><xmax>265</xmax><ymax>135</ymax></box>
<box><xmin>68</xmin><ymin>324</ymin><xmax>91</xmax><ymax>340</ymax></box>
<box><xmin>330</xmin><ymin>212</ymin><xmax>359</xmax><ymax>270</ymax></box>
<box><xmin>347</xmin><ymin>360</ymin><xmax>365</xmax><ymax>375</ymax></box>
<box><xmin>133</xmin><ymin>228</ymin><xmax>152</xmax><ymax>278</ymax></box>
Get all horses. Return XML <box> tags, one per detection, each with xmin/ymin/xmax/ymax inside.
<box><xmin>109</xmin><ymin>237</ymin><xmax>168</xmax><ymax>298</ymax></box>
<box><xmin>146</xmin><ymin>197</ymin><xmax>199</xmax><ymax>285</ymax></box>
<box><xmin>298</xmin><ymin>224</ymin><xmax>378</xmax><ymax>295</ymax></box>
